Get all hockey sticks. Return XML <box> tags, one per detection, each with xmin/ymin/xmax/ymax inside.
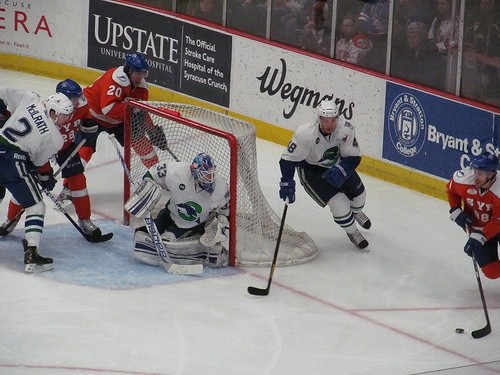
<box><xmin>464</xmin><ymin>211</ymin><xmax>491</xmax><ymax>339</ymax></box>
<box><xmin>0</xmin><ymin>138</ymin><xmax>88</xmax><ymax>237</ymax></box>
<box><xmin>34</xmin><ymin>172</ymin><xmax>114</xmax><ymax>243</ymax></box>
<box><xmin>108</xmin><ymin>134</ymin><xmax>204</xmax><ymax>274</ymax></box>
<box><xmin>166</xmin><ymin>144</ymin><xmax>181</xmax><ymax>162</ymax></box>
<box><xmin>247</xmin><ymin>197</ymin><xmax>290</xmax><ymax>296</ymax></box>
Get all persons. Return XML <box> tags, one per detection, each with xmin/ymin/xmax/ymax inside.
<box><xmin>1</xmin><ymin>78</ymin><xmax>103</xmax><ymax>236</ymax></box>
<box><xmin>54</xmin><ymin>53</ymin><xmax>167</xmax><ymax>200</ymax></box>
<box><xmin>135</xmin><ymin>153</ymin><xmax>231</xmax><ymax>267</ymax></box>
<box><xmin>447</xmin><ymin>156</ymin><xmax>500</xmax><ymax>281</ymax></box>
<box><xmin>174</xmin><ymin>0</ymin><xmax>500</xmax><ymax>103</ymax></box>
<box><xmin>278</xmin><ymin>100</ymin><xmax>371</xmax><ymax>249</ymax></box>
<box><xmin>0</xmin><ymin>88</ymin><xmax>73</xmax><ymax>264</ymax></box>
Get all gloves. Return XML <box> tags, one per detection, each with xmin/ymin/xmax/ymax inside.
<box><xmin>321</xmin><ymin>163</ymin><xmax>351</xmax><ymax>188</ymax></box>
<box><xmin>80</xmin><ymin>118</ymin><xmax>100</xmax><ymax>139</ymax></box>
<box><xmin>449</xmin><ymin>206</ymin><xmax>474</xmax><ymax>228</ymax></box>
<box><xmin>38</xmin><ymin>167</ymin><xmax>58</xmax><ymax>191</ymax></box>
<box><xmin>279</xmin><ymin>177</ymin><xmax>296</xmax><ymax>204</ymax></box>
<box><xmin>147</xmin><ymin>124</ymin><xmax>167</xmax><ymax>151</ymax></box>
<box><xmin>464</xmin><ymin>230</ymin><xmax>487</xmax><ymax>259</ymax></box>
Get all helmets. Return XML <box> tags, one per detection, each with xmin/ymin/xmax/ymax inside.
<box><xmin>191</xmin><ymin>153</ymin><xmax>216</xmax><ymax>194</ymax></box>
<box><xmin>124</xmin><ymin>53</ymin><xmax>149</xmax><ymax>72</ymax></box>
<box><xmin>46</xmin><ymin>92</ymin><xmax>74</xmax><ymax>117</ymax></box>
<box><xmin>470</xmin><ymin>152</ymin><xmax>500</xmax><ymax>172</ymax></box>
<box><xmin>56</xmin><ymin>79</ymin><xmax>82</xmax><ymax>99</ymax></box>
<box><xmin>318</xmin><ymin>100</ymin><xmax>339</xmax><ymax>117</ymax></box>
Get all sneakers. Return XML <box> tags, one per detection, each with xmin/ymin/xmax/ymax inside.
<box><xmin>53</xmin><ymin>186</ymin><xmax>72</xmax><ymax>210</ymax></box>
<box><xmin>0</xmin><ymin>215</ymin><xmax>21</xmax><ymax>237</ymax></box>
<box><xmin>354</xmin><ymin>211</ymin><xmax>372</xmax><ymax>230</ymax></box>
<box><xmin>78</xmin><ymin>218</ymin><xmax>101</xmax><ymax>237</ymax></box>
<box><xmin>22</xmin><ymin>239</ymin><xmax>54</xmax><ymax>272</ymax></box>
<box><xmin>347</xmin><ymin>229</ymin><xmax>370</xmax><ymax>252</ymax></box>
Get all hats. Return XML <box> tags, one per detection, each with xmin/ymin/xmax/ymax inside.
<box><xmin>407</xmin><ymin>21</ymin><xmax>426</xmax><ymax>34</ymax></box>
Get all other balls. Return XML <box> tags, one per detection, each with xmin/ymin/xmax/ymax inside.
<box><xmin>455</xmin><ymin>328</ymin><xmax>464</xmax><ymax>334</ymax></box>
